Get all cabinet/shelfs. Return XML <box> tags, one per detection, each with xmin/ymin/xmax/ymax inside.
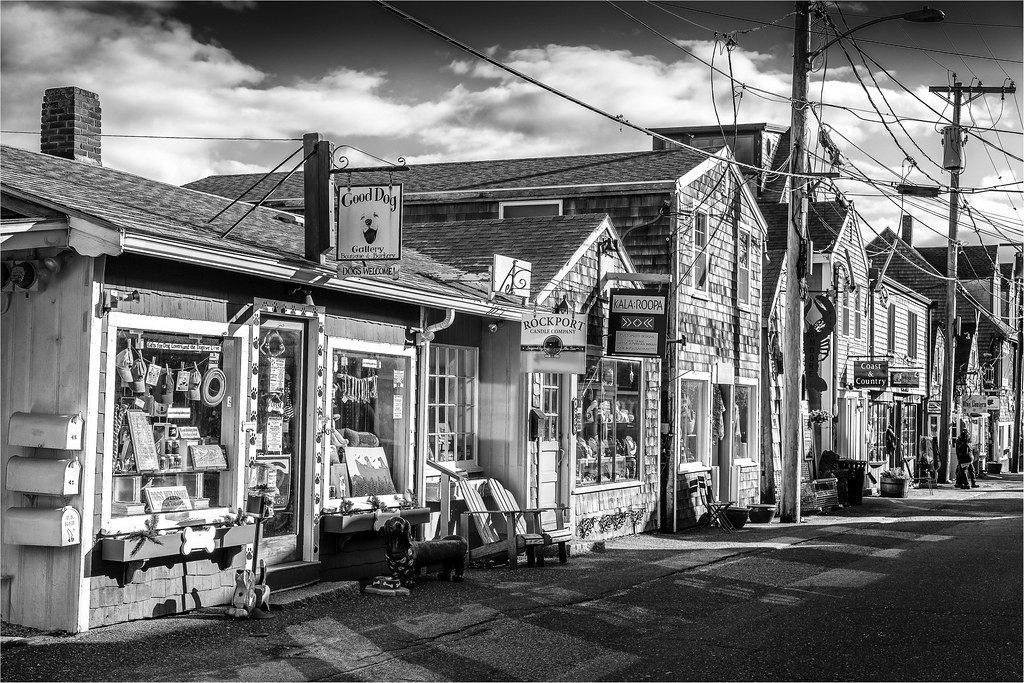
<box><xmin>577</xmin><ymin>420</ymin><xmax>635</xmax><ymax>461</ymax></box>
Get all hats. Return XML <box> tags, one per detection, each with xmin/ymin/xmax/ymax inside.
<box><xmin>153</xmin><ymin>373</ymin><xmax>174</xmax><ymax>404</ymax></box>
<box><xmin>185</xmin><ymin>368</ymin><xmax>202</xmax><ymax>401</ymax></box>
<box><xmin>116</xmin><ymin>349</ymin><xmax>134</xmax><ymax>382</ymax></box>
<box><xmin>128</xmin><ymin>358</ymin><xmax>147</xmax><ymax>393</ymax></box>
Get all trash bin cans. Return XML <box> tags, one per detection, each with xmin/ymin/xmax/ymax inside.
<box><xmin>823</xmin><ymin>469</ymin><xmax>850</xmax><ymax>504</ymax></box>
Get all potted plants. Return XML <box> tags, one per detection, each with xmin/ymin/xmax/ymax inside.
<box><xmin>325</xmin><ymin>490</ymin><xmax>432</xmax><ymax>534</ymax></box>
<box><xmin>102</xmin><ymin>507</ymin><xmax>264</xmax><ymax>563</ymax></box>
<box><xmin>880</xmin><ymin>466</ymin><xmax>910</xmax><ymax>499</ymax></box>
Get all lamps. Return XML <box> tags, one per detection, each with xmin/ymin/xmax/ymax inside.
<box><xmin>489</xmin><ymin>320</ymin><xmax>504</xmax><ymax>333</ymax></box>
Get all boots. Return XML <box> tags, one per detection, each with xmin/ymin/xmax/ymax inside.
<box><xmin>971</xmin><ymin>481</ymin><xmax>980</xmax><ymax>487</ymax></box>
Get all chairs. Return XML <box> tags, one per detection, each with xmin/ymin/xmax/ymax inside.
<box><xmin>488</xmin><ymin>478</ymin><xmax>573</xmax><ymax>568</ymax></box>
<box><xmin>902</xmin><ymin>458</ymin><xmax>934</xmax><ymax>495</ymax></box>
<box><xmin>458</xmin><ymin>479</ymin><xmax>547</xmax><ymax>571</ymax></box>
<box><xmin>704</xmin><ymin>474</ymin><xmax>738</xmax><ymax>533</ymax></box>
<box><xmin>698</xmin><ymin>473</ymin><xmax>732</xmax><ymax>534</ymax></box>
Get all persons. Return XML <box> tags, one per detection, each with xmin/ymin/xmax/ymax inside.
<box><xmin>954</xmin><ymin>429</ymin><xmax>972</xmax><ymax>487</ymax></box>
<box><xmin>958</xmin><ymin>433</ymin><xmax>979</xmax><ymax>488</ymax></box>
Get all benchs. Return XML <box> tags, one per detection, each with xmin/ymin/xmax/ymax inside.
<box><xmin>773</xmin><ymin>462</ymin><xmax>844</xmax><ymax>513</ymax></box>
